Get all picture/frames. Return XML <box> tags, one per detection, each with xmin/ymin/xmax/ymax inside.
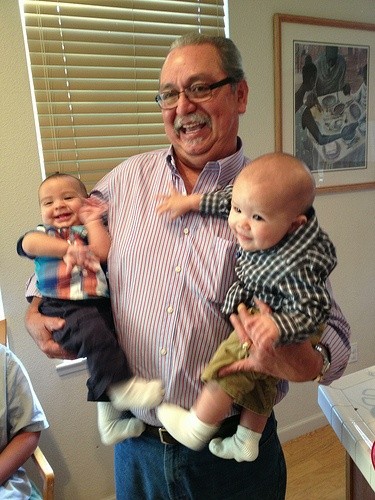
<box><xmin>273</xmin><ymin>13</ymin><xmax>375</xmax><ymax>194</ymax></box>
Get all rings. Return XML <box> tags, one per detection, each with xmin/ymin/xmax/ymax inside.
<box><xmin>242</xmin><ymin>339</ymin><xmax>252</xmax><ymax>351</ymax></box>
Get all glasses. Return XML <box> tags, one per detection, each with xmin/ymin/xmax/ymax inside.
<box><xmin>155</xmin><ymin>78</ymin><xmax>233</xmax><ymax>109</ymax></box>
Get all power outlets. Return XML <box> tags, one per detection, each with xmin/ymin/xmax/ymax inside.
<box><xmin>349</xmin><ymin>343</ymin><xmax>358</xmax><ymax>363</ymax></box>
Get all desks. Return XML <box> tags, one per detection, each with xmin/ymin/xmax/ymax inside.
<box><xmin>317</xmin><ymin>365</ymin><xmax>375</xmax><ymax>500</ymax></box>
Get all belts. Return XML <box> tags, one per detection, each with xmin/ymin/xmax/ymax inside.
<box><xmin>143</xmin><ymin>416</ymin><xmax>240</xmax><ymax>447</ymax></box>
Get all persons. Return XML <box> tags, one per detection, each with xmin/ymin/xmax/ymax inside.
<box><xmin>16</xmin><ymin>175</ymin><xmax>163</xmax><ymax>445</ymax></box>
<box><xmin>154</xmin><ymin>153</ymin><xmax>337</xmax><ymax>462</ymax></box>
<box><xmin>24</xmin><ymin>34</ymin><xmax>351</xmax><ymax>500</ymax></box>
<box><xmin>0</xmin><ymin>343</ymin><xmax>49</xmax><ymax>500</ymax></box>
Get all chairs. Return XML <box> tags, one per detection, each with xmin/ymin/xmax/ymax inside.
<box><xmin>0</xmin><ymin>320</ymin><xmax>56</xmax><ymax>500</ymax></box>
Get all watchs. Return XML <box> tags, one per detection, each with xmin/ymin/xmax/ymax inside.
<box><xmin>311</xmin><ymin>345</ymin><xmax>331</xmax><ymax>384</ymax></box>
